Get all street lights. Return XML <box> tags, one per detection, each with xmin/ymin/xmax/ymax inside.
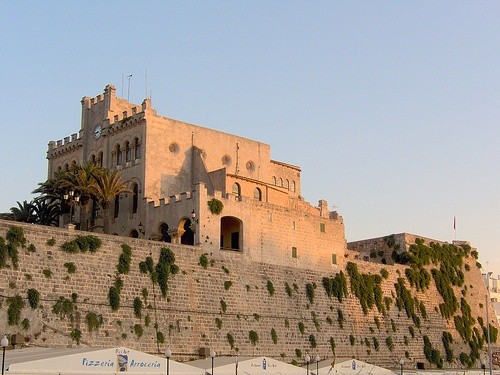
<box><xmin>63</xmin><ymin>188</ymin><xmax>80</xmax><ymax>231</ymax></box>
<box><xmin>305</xmin><ymin>354</ymin><xmax>311</xmax><ymax>375</ymax></box>
<box><xmin>316</xmin><ymin>355</ymin><xmax>321</xmax><ymax>375</ymax></box>
<box><xmin>400</xmin><ymin>358</ymin><xmax>404</xmax><ymax>375</ymax></box>
<box><xmin>164</xmin><ymin>348</ymin><xmax>172</xmax><ymax>374</ymax></box>
<box><xmin>210</xmin><ymin>350</ymin><xmax>216</xmax><ymax>375</ymax></box>
<box><xmin>1</xmin><ymin>333</ymin><xmax>9</xmax><ymax>375</ymax></box>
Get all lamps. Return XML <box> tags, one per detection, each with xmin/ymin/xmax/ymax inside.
<box><xmin>191</xmin><ymin>209</ymin><xmax>196</xmax><ymax>220</ymax></box>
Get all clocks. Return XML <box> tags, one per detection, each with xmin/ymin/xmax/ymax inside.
<box><xmin>94</xmin><ymin>125</ymin><xmax>101</xmax><ymax>140</ymax></box>
